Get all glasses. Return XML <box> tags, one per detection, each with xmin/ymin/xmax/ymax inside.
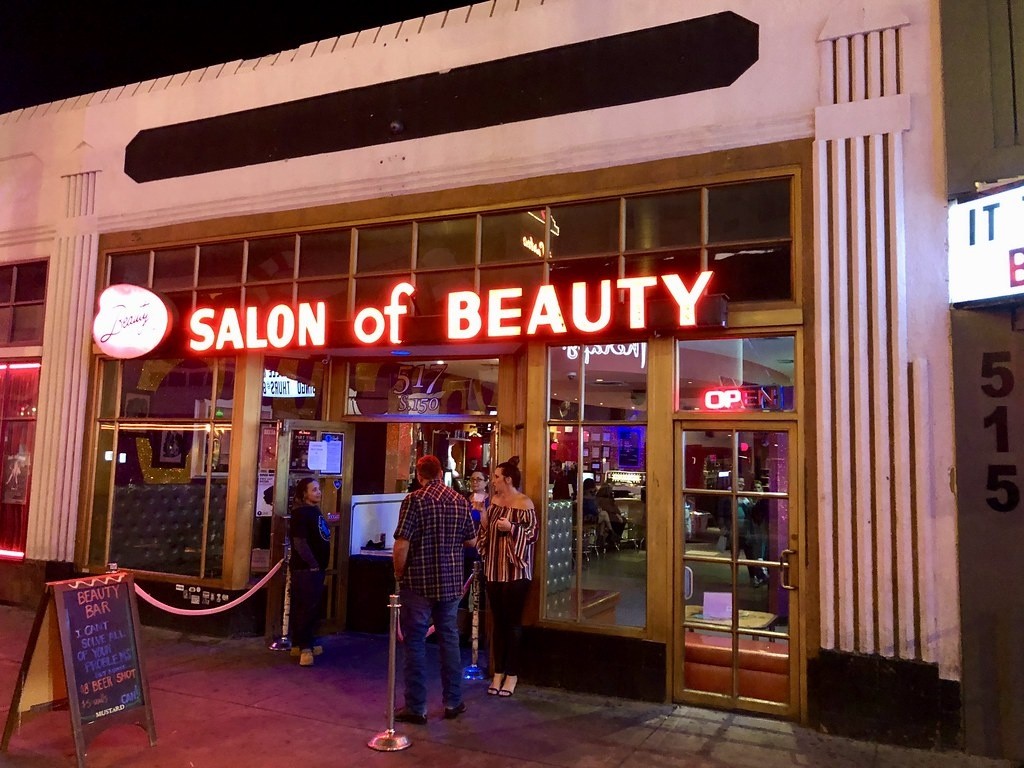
<box><xmin>470</xmin><ymin>478</ymin><xmax>486</xmax><ymax>483</ymax></box>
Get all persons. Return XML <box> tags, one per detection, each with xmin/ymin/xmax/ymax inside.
<box><xmin>552</xmin><ymin>460</ymin><xmax>624</xmax><ymax>552</ymax></box>
<box><xmin>289</xmin><ymin>477</ymin><xmax>331</xmax><ymax>665</ymax></box>
<box><xmin>715</xmin><ymin>475</ymin><xmax>769</xmax><ymax>588</ymax></box>
<box><xmin>385</xmin><ymin>455</ymin><xmax>539</xmax><ymax>726</ymax></box>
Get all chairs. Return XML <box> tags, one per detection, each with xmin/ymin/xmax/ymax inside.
<box><xmin>571</xmin><ymin>498</ymin><xmax>600</xmax><ymax>561</ymax></box>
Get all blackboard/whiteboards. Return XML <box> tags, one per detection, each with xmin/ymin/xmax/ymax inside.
<box><xmin>53</xmin><ymin>572</ymin><xmax>152</xmax><ymax>724</ymax></box>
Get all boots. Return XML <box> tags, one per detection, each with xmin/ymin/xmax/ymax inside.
<box><xmin>608</xmin><ymin>531</ymin><xmax>622</xmax><ymax>543</ymax></box>
<box><xmin>595</xmin><ymin>535</ymin><xmax>604</xmax><ymax>547</ymax></box>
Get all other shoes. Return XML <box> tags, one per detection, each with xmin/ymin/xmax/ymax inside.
<box><xmin>300</xmin><ymin>652</ymin><xmax>314</xmax><ymax>666</ymax></box>
<box><xmin>395</xmin><ymin>706</ymin><xmax>427</xmax><ymax>724</ymax></box>
<box><xmin>444</xmin><ymin>703</ymin><xmax>466</xmax><ymax>719</ymax></box>
<box><xmin>290</xmin><ymin>645</ymin><xmax>324</xmax><ymax>656</ymax></box>
<box><xmin>750</xmin><ymin>577</ymin><xmax>760</xmax><ymax>587</ymax></box>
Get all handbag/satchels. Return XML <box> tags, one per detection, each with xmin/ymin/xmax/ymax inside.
<box><xmin>717</xmin><ymin>535</ymin><xmax>730</xmax><ymax>552</ymax></box>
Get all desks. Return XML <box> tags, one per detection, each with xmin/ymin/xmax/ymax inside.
<box><xmin>684</xmin><ymin>605</ymin><xmax>778</xmax><ymax>643</ymax></box>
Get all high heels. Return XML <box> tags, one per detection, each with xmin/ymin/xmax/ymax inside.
<box><xmin>487</xmin><ymin>678</ymin><xmax>518</xmax><ymax>697</ymax></box>
